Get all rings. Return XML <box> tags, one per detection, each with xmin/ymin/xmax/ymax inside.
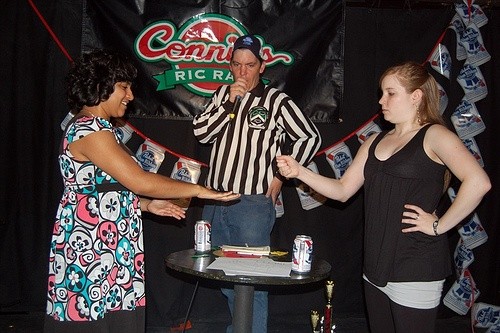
<box><xmin>416</xmin><ymin>214</ymin><xmax>419</xmax><ymax>219</ymax></box>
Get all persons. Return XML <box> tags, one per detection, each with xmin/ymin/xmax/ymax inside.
<box><xmin>274</xmin><ymin>63</ymin><xmax>492</xmax><ymax>333</ymax></box>
<box><xmin>43</xmin><ymin>49</ymin><xmax>241</xmax><ymax>333</ymax></box>
<box><xmin>193</xmin><ymin>36</ymin><xmax>322</xmax><ymax>333</ymax></box>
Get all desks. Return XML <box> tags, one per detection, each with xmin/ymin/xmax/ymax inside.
<box><xmin>165</xmin><ymin>246</ymin><xmax>331</xmax><ymax>333</ymax></box>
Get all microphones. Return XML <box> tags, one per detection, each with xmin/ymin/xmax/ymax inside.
<box><xmin>230</xmin><ymin>95</ymin><xmax>240</xmax><ymax>122</ymax></box>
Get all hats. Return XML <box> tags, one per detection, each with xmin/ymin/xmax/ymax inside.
<box><xmin>232</xmin><ymin>35</ymin><xmax>262</xmax><ymax>61</ymax></box>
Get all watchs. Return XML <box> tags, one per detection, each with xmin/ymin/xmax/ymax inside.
<box><xmin>433</xmin><ymin>219</ymin><xmax>438</xmax><ymax>236</ymax></box>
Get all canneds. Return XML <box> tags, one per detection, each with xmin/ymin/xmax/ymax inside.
<box><xmin>292</xmin><ymin>234</ymin><xmax>313</xmax><ymax>274</ymax></box>
<box><xmin>194</xmin><ymin>220</ymin><xmax>212</xmax><ymax>253</ymax></box>
